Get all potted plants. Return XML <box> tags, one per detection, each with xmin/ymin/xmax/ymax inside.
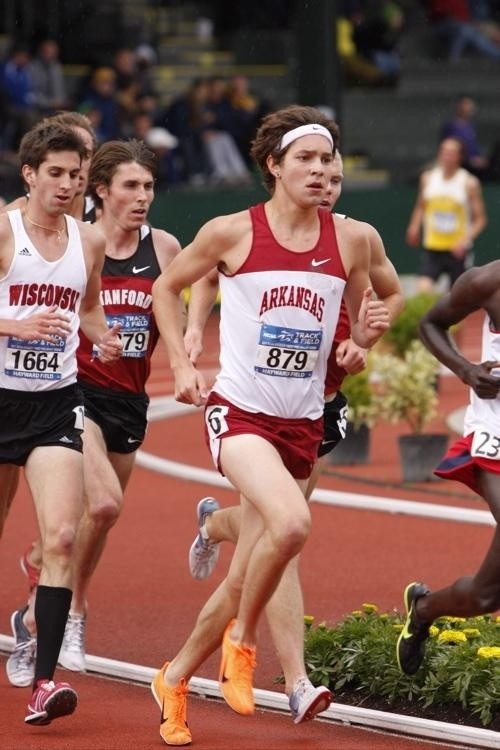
<box><xmin>371</xmin><ymin>339</ymin><xmax>453</xmax><ymax>484</ymax></box>
<box><xmin>390</xmin><ymin>292</ymin><xmax>457</xmax><ymax>394</ymax></box>
<box><xmin>330</xmin><ymin>367</ymin><xmax>375</xmax><ymax>466</ymax></box>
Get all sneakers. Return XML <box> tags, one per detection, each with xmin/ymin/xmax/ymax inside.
<box><xmin>396</xmin><ymin>583</ymin><xmax>433</xmax><ymax>675</ymax></box>
<box><xmin>151</xmin><ymin>661</ymin><xmax>191</xmax><ymax>746</ymax></box>
<box><xmin>219</xmin><ymin>618</ymin><xmax>256</xmax><ymax>715</ymax></box>
<box><xmin>6</xmin><ymin>547</ymin><xmax>86</xmax><ymax>726</ymax></box>
<box><xmin>188</xmin><ymin>497</ymin><xmax>219</xmax><ymax>580</ymax></box>
<box><xmin>289</xmin><ymin>682</ymin><xmax>331</xmax><ymax>724</ymax></box>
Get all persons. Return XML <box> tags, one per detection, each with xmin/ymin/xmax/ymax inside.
<box><xmin>404</xmin><ymin>94</ymin><xmax>498</xmax><ymax>286</ymax></box>
<box><xmin>6</xmin><ymin>146</ymin><xmax>181</xmax><ymax>688</ymax></box>
<box><xmin>1</xmin><ymin>2</ymin><xmax>294</xmax><ymax>189</ymax></box>
<box><xmin>396</xmin><ymin>261</ymin><xmax>500</xmax><ymax>677</ymax></box>
<box><xmin>184</xmin><ymin>146</ymin><xmax>408</xmax><ymax>723</ymax></box>
<box><xmin>151</xmin><ymin>109</ymin><xmax>390</xmax><ymax>746</ymax></box>
<box><xmin>0</xmin><ymin>122</ymin><xmax>122</xmax><ymax>726</ymax></box>
<box><xmin>6</xmin><ymin>116</ymin><xmax>99</xmax><ymax>588</ymax></box>
<box><xmin>335</xmin><ymin>1</ymin><xmax>498</xmax><ymax>85</ymax></box>
